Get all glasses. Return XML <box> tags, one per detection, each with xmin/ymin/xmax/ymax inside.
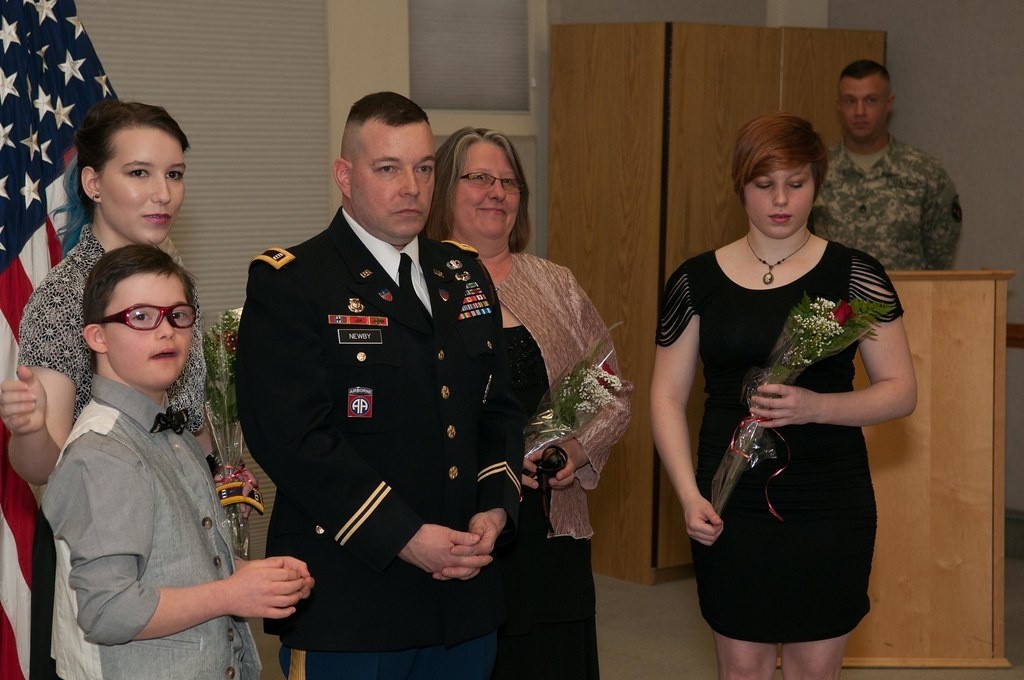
<box><xmin>82</xmin><ymin>302</ymin><xmax>198</xmax><ymax>331</ymax></box>
<box><xmin>460</xmin><ymin>172</ymin><xmax>524</xmax><ymax>192</ymax></box>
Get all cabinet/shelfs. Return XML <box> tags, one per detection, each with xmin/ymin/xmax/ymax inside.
<box><xmin>544</xmin><ymin>18</ymin><xmax>889</xmax><ymax>583</ymax></box>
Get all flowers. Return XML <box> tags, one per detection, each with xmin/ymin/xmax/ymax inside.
<box><xmin>204</xmin><ymin>307</ymin><xmax>252</xmax><ymax>561</ymax></box>
<box><xmin>527</xmin><ymin>320</ymin><xmax>636</xmax><ymax>445</ymax></box>
<box><xmin>714</xmin><ymin>292</ymin><xmax>896</xmax><ymax>516</ymax></box>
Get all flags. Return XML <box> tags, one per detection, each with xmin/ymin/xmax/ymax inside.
<box><xmin>0</xmin><ymin>0</ymin><xmax>118</xmax><ymax>680</ymax></box>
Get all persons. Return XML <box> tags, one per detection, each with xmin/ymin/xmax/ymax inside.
<box><xmin>0</xmin><ymin>91</ymin><xmax>631</xmax><ymax>680</ymax></box>
<box><xmin>805</xmin><ymin>60</ymin><xmax>963</xmax><ymax>272</ymax></box>
<box><xmin>654</xmin><ymin>109</ymin><xmax>919</xmax><ymax>680</ymax></box>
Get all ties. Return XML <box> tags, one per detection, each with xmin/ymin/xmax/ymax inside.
<box><xmin>398</xmin><ymin>253</ymin><xmax>435</xmax><ymax>327</ymax></box>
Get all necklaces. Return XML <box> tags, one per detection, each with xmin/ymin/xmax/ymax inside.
<box><xmin>746</xmin><ymin>231</ymin><xmax>812</xmax><ymax>285</ymax></box>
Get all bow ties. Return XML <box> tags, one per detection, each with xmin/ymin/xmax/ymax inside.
<box><xmin>149</xmin><ymin>408</ymin><xmax>188</xmax><ymax>435</ymax></box>
